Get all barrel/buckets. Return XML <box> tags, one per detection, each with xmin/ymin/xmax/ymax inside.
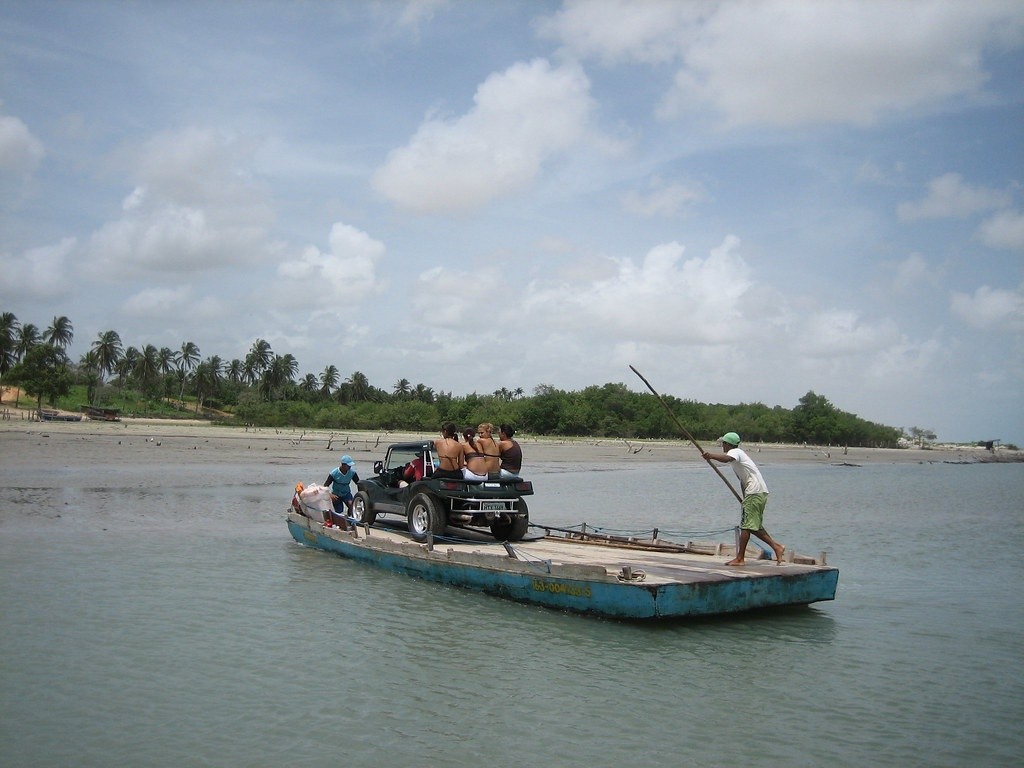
<box><xmin>301</xmin><ymin>484</ymin><xmax>334</xmax><ymax>524</ymax></box>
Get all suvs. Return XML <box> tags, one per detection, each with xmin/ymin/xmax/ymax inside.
<box><xmin>352</xmin><ymin>440</ymin><xmax>534</xmax><ymax>543</ymax></box>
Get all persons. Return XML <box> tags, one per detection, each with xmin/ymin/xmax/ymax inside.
<box><xmin>701</xmin><ymin>432</ymin><xmax>786</xmax><ymax>566</ymax></box>
<box><xmin>477</xmin><ymin>423</ymin><xmax>501</xmax><ymax>479</ymax></box>
<box><xmin>460</xmin><ymin>428</ymin><xmax>488</xmax><ymax>481</ymax></box>
<box><xmin>497</xmin><ymin>424</ymin><xmax>522</xmax><ymax>476</ymax></box>
<box><xmin>398</xmin><ymin>451</ymin><xmax>424</xmax><ymax>487</ymax></box>
<box><xmin>323</xmin><ymin>455</ymin><xmax>359</xmax><ymax>518</ymax></box>
<box><xmin>432</xmin><ymin>422</ymin><xmax>464</xmax><ymax>479</ymax></box>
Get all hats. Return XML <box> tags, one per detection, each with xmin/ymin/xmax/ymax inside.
<box><xmin>716</xmin><ymin>432</ymin><xmax>741</xmax><ymax>445</ymax></box>
<box><xmin>341</xmin><ymin>455</ymin><xmax>355</xmax><ymax>466</ymax></box>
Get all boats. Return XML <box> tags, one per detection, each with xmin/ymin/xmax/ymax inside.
<box><xmin>39</xmin><ymin>410</ymin><xmax>82</xmax><ymax>421</ymax></box>
<box><xmin>285</xmin><ymin>507</ymin><xmax>838</xmax><ymax>623</ymax></box>
<box><xmin>82</xmin><ymin>402</ymin><xmax>121</xmax><ymax>422</ymax></box>
